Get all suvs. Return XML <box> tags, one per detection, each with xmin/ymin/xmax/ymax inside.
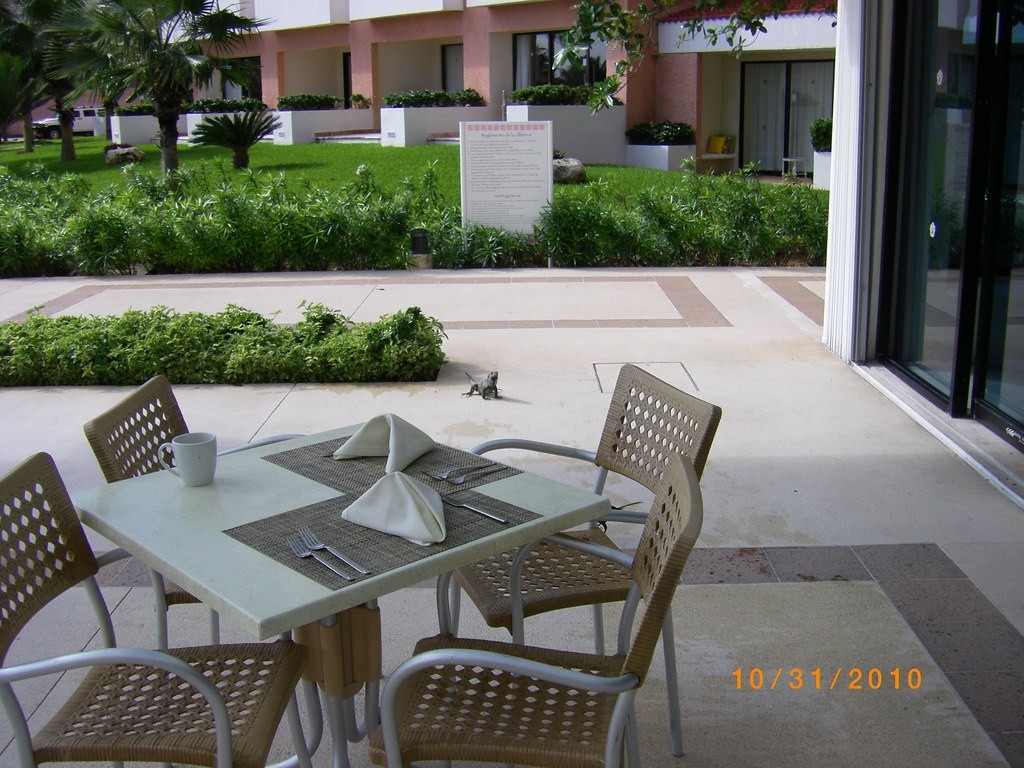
<box><xmin>32</xmin><ymin>106</ymin><xmax>106</xmax><ymax>140</ymax></box>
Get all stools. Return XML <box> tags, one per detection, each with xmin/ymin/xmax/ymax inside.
<box><xmin>781</xmin><ymin>157</ymin><xmax>807</xmax><ymax>180</ymax></box>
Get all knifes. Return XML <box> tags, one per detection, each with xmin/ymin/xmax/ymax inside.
<box><xmin>440</xmin><ymin>494</ymin><xmax>509</xmax><ymax>525</ymax></box>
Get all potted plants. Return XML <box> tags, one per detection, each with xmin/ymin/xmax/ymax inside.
<box><xmin>350</xmin><ymin>93</ymin><xmax>364</xmax><ymax>109</ymax></box>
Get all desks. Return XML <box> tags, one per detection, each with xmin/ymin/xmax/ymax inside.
<box><xmin>66</xmin><ymin>414</ymin><xmax>611</xmax><ymax>768</ymax></box>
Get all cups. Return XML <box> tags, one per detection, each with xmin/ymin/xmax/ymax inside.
<box><xmin>159</xmin><ymin>432</ymin><xmax>217</xmax><ymax>486</ymax></box>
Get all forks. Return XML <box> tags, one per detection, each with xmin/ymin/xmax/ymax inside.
<box><xmin>299</xmin><ymin>525</ymin><xmax>372</xmax><ymax>575</ymax></box>
<box><xmin>419</xmin><ymin>461</ymin><xmax>499</xmax><ymax>481</ymax></box>
<box><xmin>287</xmin><ymin>534</ymin><xmax>356</xmax><ymax>581</ymax></box>
<box><xmin>439</xmin><ymin>465</ymin><xmax>511</xmax><ymax>484</ymax></box>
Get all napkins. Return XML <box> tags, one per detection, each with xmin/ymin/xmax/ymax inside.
<box><xmin>341</xmin><ymin>472</ymin><xmax>447</xmax><ymax>548</ymax></box>
<box><xmin>332</xmin><ymin>415</ymin><xmax>436</xmax><ymax>474</ymax></box>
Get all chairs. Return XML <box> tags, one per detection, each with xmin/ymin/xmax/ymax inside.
<box><xmin>83</xmin><ymin>376</ymin><xmax>326</xmax><ymax>768</ymax></box>
<box><xmin>0</xmin><ymin>452</ymin><xmax>311</xmax><ymax>768</ymax></box>
<box><xmin>452</xmin><ymin>361</ymin><xmax>723</xmax><ymax>757</ymax></box>
<box><xmin>368</xmin><ymin>452</ymin><xmax>705</xmax><ymax>768</ymax></box>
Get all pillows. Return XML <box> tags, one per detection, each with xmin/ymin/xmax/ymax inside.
<box><xmin>708</xmin><ymin>136</ymin><xmax>736</xmax><ymax>154</ymax></box>
